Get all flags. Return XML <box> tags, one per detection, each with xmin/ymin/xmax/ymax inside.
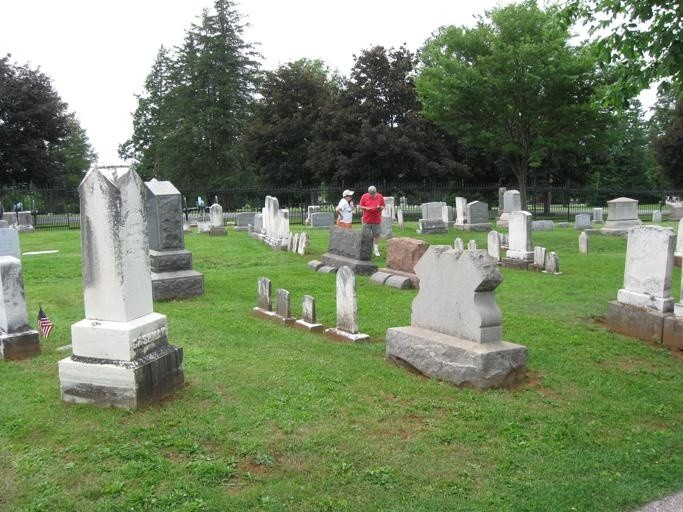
<box><xmin>37</xmin><ymin>308</ymin><xmax>53</xmax><ymax>339</ymax></box>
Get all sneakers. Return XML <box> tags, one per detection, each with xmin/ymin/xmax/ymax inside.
<box><xmin>374</xmin><ymin>250</ymin><xmax>380</xmax><ymax>257</ymax></box>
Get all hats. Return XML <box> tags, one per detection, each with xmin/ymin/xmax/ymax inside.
<box><xmin>342</xmin><ymin>189</ymin><xmax>354</xmax><ymax>197</ymax></box>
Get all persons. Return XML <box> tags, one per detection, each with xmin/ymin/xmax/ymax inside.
<box><xmin>358</xmin><ymin>185</ymin><xmax>386</xmax><ymax>257</ymax></box>
<box><xmin>196</xmin><ymin>196</ymin><xmax>206</xmax><ymax>219</ymax></box>
<box><xmin>336</xmin><ymin>189</ymin><xmax>354</xmax><ymax>229</ymax></box>
